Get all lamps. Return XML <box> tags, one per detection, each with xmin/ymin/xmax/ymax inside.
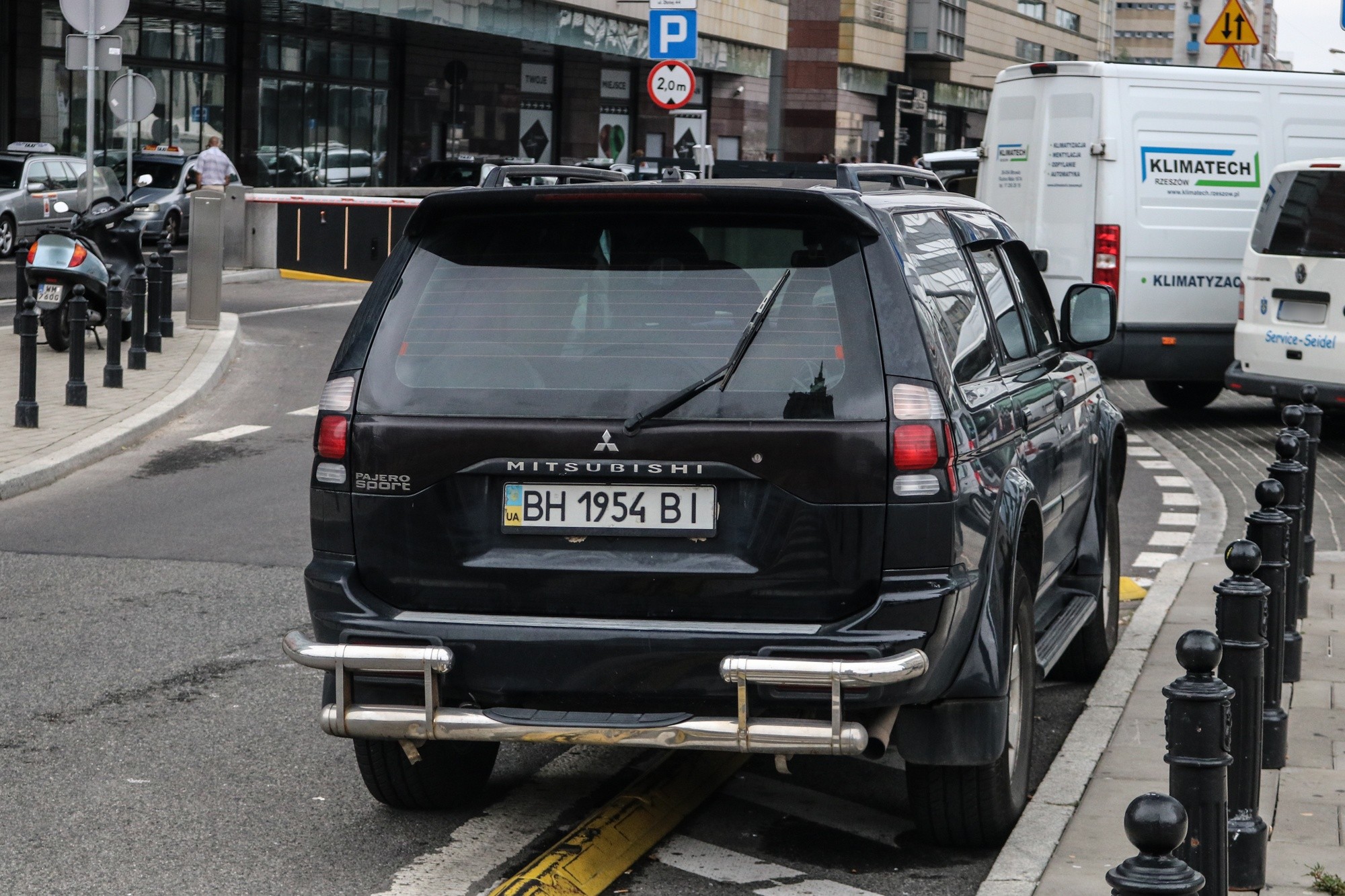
<box><xmin>736</xmin><ymin>86</ymin><xmax>744</xmax><ymax>93</ymax></box>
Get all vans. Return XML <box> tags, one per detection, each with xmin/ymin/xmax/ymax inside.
<box><xmin>914</xmin><ymin>61</ymin><xmax>1344</xmax><ymax>415</ymax></box>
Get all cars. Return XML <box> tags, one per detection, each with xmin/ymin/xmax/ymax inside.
<box><xmin>253</xmin><ymin>140</ymin><xmax>374</xmax><ymax>187</ymax></box>
<box><xmin>551</xmin><ymin>159</ymin><xmax>637</xmax><ymax>184</ymax></box>
<box><xmin>0</xmin><ymin>141</ymin><xmax>200</xmax><ymax>258</ymax></box>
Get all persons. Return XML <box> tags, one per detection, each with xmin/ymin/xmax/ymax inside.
<box><xmin>631</xmin><ymin>149</ymin><xmax>649</xmax><ymax>168</ymax></box>
<box><xmin>816</xmin><ymin>154</ymin><xmax>923</xmax><ymax>173</ymax></box>
<box><xmin>191</xmin><ymin>136</ymin><xmax>232</xmax><ymax>194</ymax></box>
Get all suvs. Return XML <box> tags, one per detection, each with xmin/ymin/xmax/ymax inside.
<box><xmin>283</xmin><ymin>161</ymin><xmax>1129</xmax><ymax>847</ymax></box>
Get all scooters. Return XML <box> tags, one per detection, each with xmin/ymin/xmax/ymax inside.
<box><xmin>23</xmin><ymin>166</ymin><xmax>153</xmax><ymax>353</ymax></box>
